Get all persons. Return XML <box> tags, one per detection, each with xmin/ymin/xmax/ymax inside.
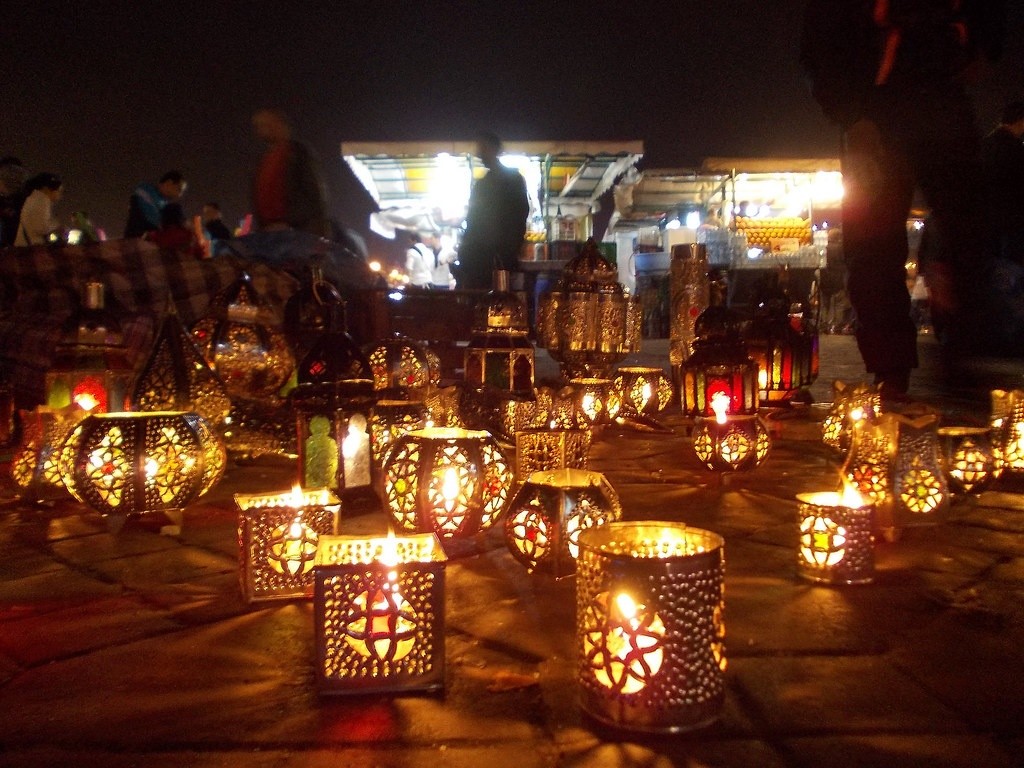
<box><xmin>200</xmin><ymin>203</ymin><xmax>235</xmax><ymax>242</ymax></box>
<box><xmin>405</xmin><ymin>233</ymin><xmax>462</xmax><ymax>290</ymax></box>
<box><xmin>801</xmin><ymin>0</ymin><xmax>985</xmax><ymax>398</ymax></box>
<box><xmin>734</xmin><ymin>199</ymin><xmax>752</xmax><ymax>218</ymax></box>
<box><xmin>985</xmin><ymin>95</ymin><xmax>1024</xmax><ymax>357</ymax></box>
<box><xmin>122</xmin><ymin>172</ymin><xmax>188</xmax><ymax>242</ymax></box>
<box><xmin>0</xmin><ymin>155</ymin><xmax>65</xmax><ymax>249</ymax></box>
<box><xmin>460</xmin><ymin>129</ymin><xmax>529</xmax><ymax>269</ymax></box>
<box><xmin>248</xmin><ymin>105</ymin><xmax>333</xmax><ymax>236</ymax></box>
